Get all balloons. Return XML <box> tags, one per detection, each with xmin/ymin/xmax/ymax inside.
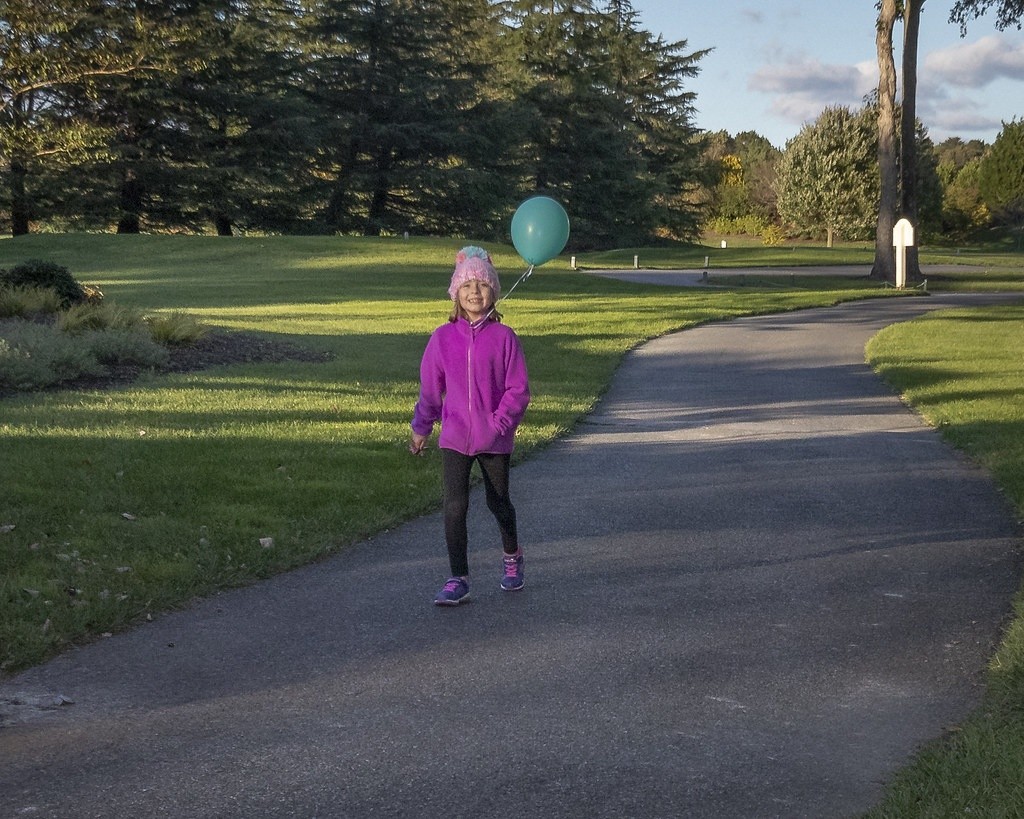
<box><xmin>510</xmin><ymin>196</ymin><xmax>570</xmax><ymax>268</ymax></box>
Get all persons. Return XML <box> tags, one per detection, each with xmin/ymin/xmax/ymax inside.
<box><xmin>408</xmin><ymin>245</ymin><xmax>527</xmax><ymax>607</ymax></box>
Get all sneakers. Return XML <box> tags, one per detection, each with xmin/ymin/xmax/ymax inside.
<box><xmin>435</xmin><ymin>579</ymin><xmax>471</xmax><ymax>606</ymax></box>
<box><xmin>501</xmin><ymin>543</ymin><xmax>525</xmax><ymax>591</ymax></box>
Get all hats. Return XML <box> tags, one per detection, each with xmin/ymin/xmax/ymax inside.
<box><xmin>447</xmin><ymin>245</ymin><xmax>500</xmax><ymax>302</ymax></box>
